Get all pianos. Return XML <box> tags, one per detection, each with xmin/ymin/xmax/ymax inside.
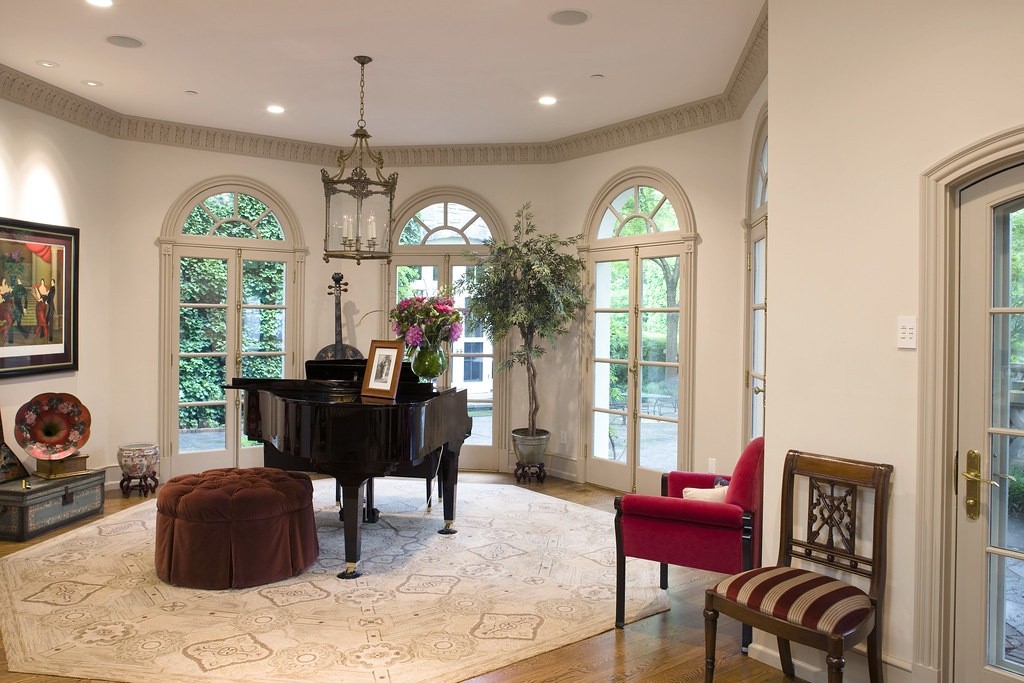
<box><xmin>230</xmin><ymin>359</ymin><xmax>477</xmax><ymax>580</ymax></box>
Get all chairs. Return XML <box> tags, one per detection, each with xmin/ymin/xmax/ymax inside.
<box><xmin>614</xmin><ymin>437</ymin><xmax>764</xmax><ymax>629</ymax></box>
<box><xmin>703</xmin><ymin>449</ymin><xmax>894</xmax><ymax>683</ymax></box>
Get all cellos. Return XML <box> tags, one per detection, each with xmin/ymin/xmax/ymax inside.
<box><xmin>316</xmin><ymin>273</ymin><xmax>364</xmax><ymax>359</ymax></box>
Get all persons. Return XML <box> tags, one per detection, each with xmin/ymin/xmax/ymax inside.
<box><xmin>379</xmin><ymin>357</ymin><xmax>388</xmax><ymax>380</ymax></box>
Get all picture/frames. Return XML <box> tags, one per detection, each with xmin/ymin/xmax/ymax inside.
<box><xmin>0</xmin><ymin>217</ymin><xmax>80</xmax><ymax>378</ymax></box>
<box><xmin>359</xmin><ymin>340</ymin><xmax>406</xmax><ymax>399</ymax></box>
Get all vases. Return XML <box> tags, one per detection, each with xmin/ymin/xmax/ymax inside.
<box><xmin>117</xmin><ymin>442</ymin><xmax>159</xmax><ymax>476</ymax></box>
<box><xmin>411</xmin><ymin>344</ymin><xmax>448</xmax><ymax>383</ymax></box>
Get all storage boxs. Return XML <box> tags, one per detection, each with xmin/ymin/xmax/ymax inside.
<box><xmin>0</xmin><ymin>469</ymin><xmax>105</xmax><ymax>541</ymax></box>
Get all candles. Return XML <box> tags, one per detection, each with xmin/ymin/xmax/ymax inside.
<box><xmin>342</xmin><ymin>211</ymin><xmax>378</xmax><ymax>241</ymax></box>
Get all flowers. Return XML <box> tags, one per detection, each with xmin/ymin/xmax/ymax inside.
<box><xmin>389</xmin><ymin>298</ymin><xmax>462</xmax><ymax>357</ymax></box>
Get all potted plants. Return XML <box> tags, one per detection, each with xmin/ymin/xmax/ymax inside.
<box><xmin>451</xmin><ymin>199</ymin><xmax>591</xmax><ymax>483</ymax></box>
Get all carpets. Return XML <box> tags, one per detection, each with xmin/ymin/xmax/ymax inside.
<box><xmin>0</xmin><ymin>477</ymin><xmax>671</xmax><ymax>682</ymax></box>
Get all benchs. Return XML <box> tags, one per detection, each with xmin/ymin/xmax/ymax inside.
<box><xmin>621</xmin><ymin>392</ymin><xmax>676</xmax><ymax>416</ymax></box>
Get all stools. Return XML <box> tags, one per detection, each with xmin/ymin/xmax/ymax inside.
<box><xmin>155</xmin><ymin>467</ymin><xmax>319</xmax><ymax>590</ymax></box>
